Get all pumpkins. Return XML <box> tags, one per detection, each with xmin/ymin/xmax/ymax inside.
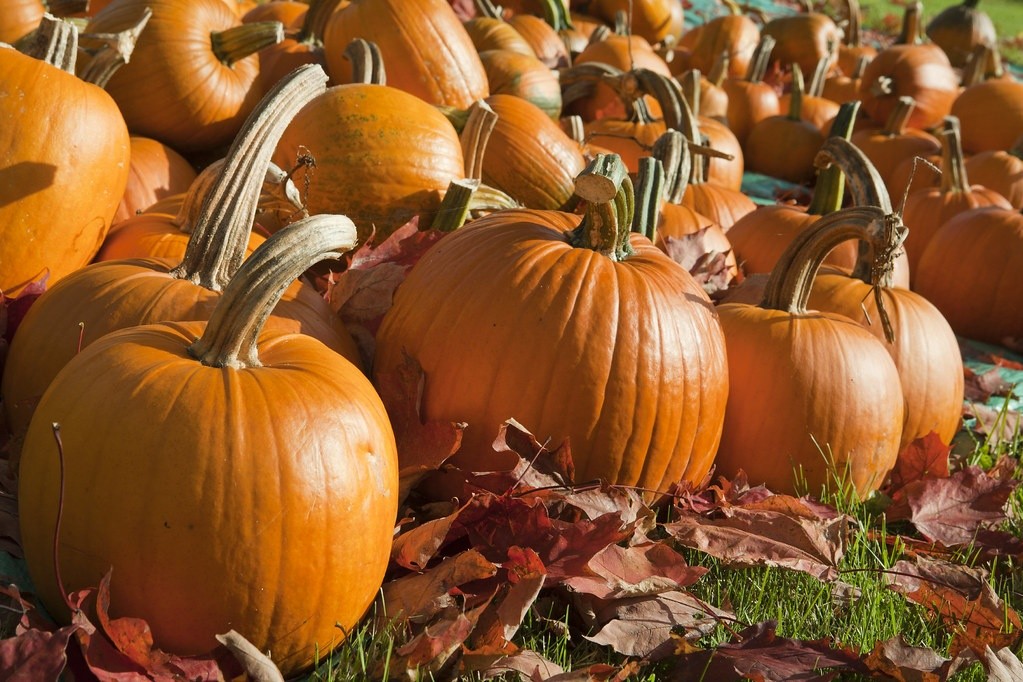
<box><xmin>0</xmin><ymin>1</ymin><xmax>1023</xmax><ymax>682</ymax></box>
